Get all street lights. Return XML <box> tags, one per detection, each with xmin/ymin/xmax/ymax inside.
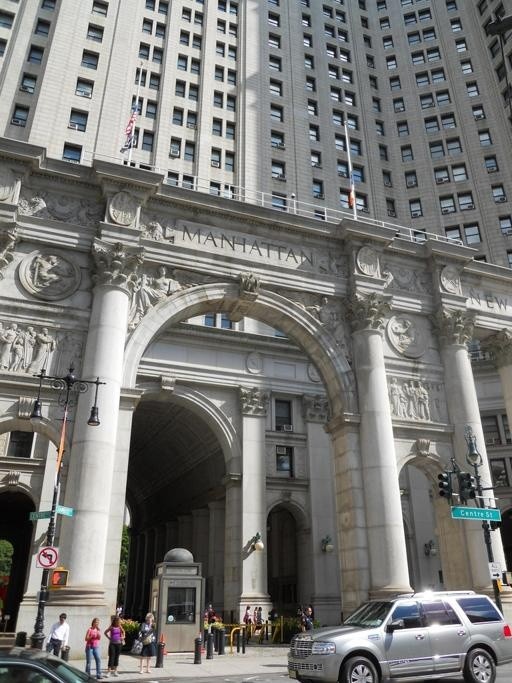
<box><xmin>29</xmin><ymin>361</ymin><xmax>101</xmax><ymax>647</ymax></box>
<box><xmin>461</xmin><ymin>431</ymin><xmax>503</xmax><ymax>619</ymax></box>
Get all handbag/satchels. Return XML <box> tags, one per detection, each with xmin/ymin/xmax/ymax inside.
<box><xmin>131</xmin><ymin>640</ymin><xmax>142</xmax><ymax>654</ymax></box>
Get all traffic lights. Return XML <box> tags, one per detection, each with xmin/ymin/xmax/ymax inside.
<box><xmin>52</xmin><ymin>568</ymin><xmax>69</xmax><ymax>590</ymax></box>
<box><xmin>440</xmin><ymin>470</ymin><xmax>452</xmax><ymax>496</ymax></box>
<box><xmin>461</xmin><ymin>472</ymin><xmax>471</xmax><ymax>500</ymax></box>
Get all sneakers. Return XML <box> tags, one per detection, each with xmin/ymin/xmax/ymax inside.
<box><xmin>96</xmin><ymin>672</ymin><xmax>118</xmax><ymax>679</ymax></box>
<box><xmin>140</xmin><ymin>671</ymin><xmax>150</xmax><ymax>674</ymax></box>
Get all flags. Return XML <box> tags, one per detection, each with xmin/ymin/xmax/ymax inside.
<box><xmin>125</xmin><ymin>104</ymin><xmax>139</xmax><ymax>139</ymax></box>
<box><xmin>347</xmin><ymin>167</ymin><xmax>354</xmax><ymax>207</ymax></box>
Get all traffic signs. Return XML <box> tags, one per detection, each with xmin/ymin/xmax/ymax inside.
<box><xmin>489</xmin><ymin>562</ymin><xmax>502</xmax><ymax>580</ymax></box>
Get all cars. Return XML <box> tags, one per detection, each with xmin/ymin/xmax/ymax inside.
<box><xmin>0</xmin><ymin>646</ymin><xmax>102</xmax><ymax>683</ymax></box>
<box><xmin>287</xmin><ymin>588</ymin><xmax>512</xmax><ymax>683</ymax></box>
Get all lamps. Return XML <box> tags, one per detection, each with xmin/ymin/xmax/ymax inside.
<box><xmin>252</xmin><ymin>532</ymin><xmax>265</xmax><ymax>551</ymax></box>
<box><xmin>321</xmin><ymin>535</ymin><xmax>334</xmax><ymax>552</ymax></box>
<box><xmin>424</xmin><ymin>539</ymin><xmax>437</xmax><ymax>557</ymax></box>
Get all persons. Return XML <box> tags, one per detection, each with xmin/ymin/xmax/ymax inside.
<box><xmin>35</xmin><ymin>256</ymin><xmax>63</xmax><ymax>286</ymax></box>
<box><xmin>44</xmin><ymin>613</ymin><xmax>69</xmax><ymax>657</ymax></box>
<box><xmin>104</xmin><ymin>617</ymin><xmax>126</xmax><ymax>677</ymax></box>
<box><xmin>203</xmin><ymin>603</ymin><xmax>264</xmax><ymax>648</ymax></box>
<box><xmin>305</xmin><ymin>297</ymin><xmax>346</xmax><ymax>347</ymax></box>
<box><xmin>301</xmin><ymin>607</ymin><xmax>313</xmax><ymax>633</ymax></box>
<box><xmin>84</xmin><ymin>618</ymin><xmax>105</xmax><ymax>679</ymax></box>
<box><xmin>0</xmin><ymin>323</ymin><xmax>82</xmax><ymax>377</ymax></box>
<box><xmin>389</xmin><ymin>377</ymin><xmax>431</xmax><ymax>422</ymax></box>
<box><xmin>136</xmin><ymin>612</ymin><xmax>156</xmax><ymax>674</ymax></box>
<box><xmin>392</xmin><ymin>319</ymin><xmax>418</xmax><ymax>351</ymax></box>
<box><xmin>128</xmin><ymin>264</ymin><xmax>181</xmax><ymax>332</ymax></box>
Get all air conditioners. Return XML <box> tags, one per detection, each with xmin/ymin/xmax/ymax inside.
<box><xmin>170</xmin><ymin>122</ymin><xmax>221</xmax><ymax>167</ymax></box>
<box><xmin>383</xmin><ymin>166</ymin><xmax>512</xmax><ymax>236</ymax></box>
<box><xmin>67</xmin><ymin>90</ymin><xmax>91</xmax><ymax>130</ymax></box>
<box><xmin>476</xmin><ymin>113</ymin><xmax>485</xmax><ymax>119</ymax></box>
<box><xmin>276</xmin><ymin>141</ymin><xmax>369</xmax><ymax>212</ymax></box>
